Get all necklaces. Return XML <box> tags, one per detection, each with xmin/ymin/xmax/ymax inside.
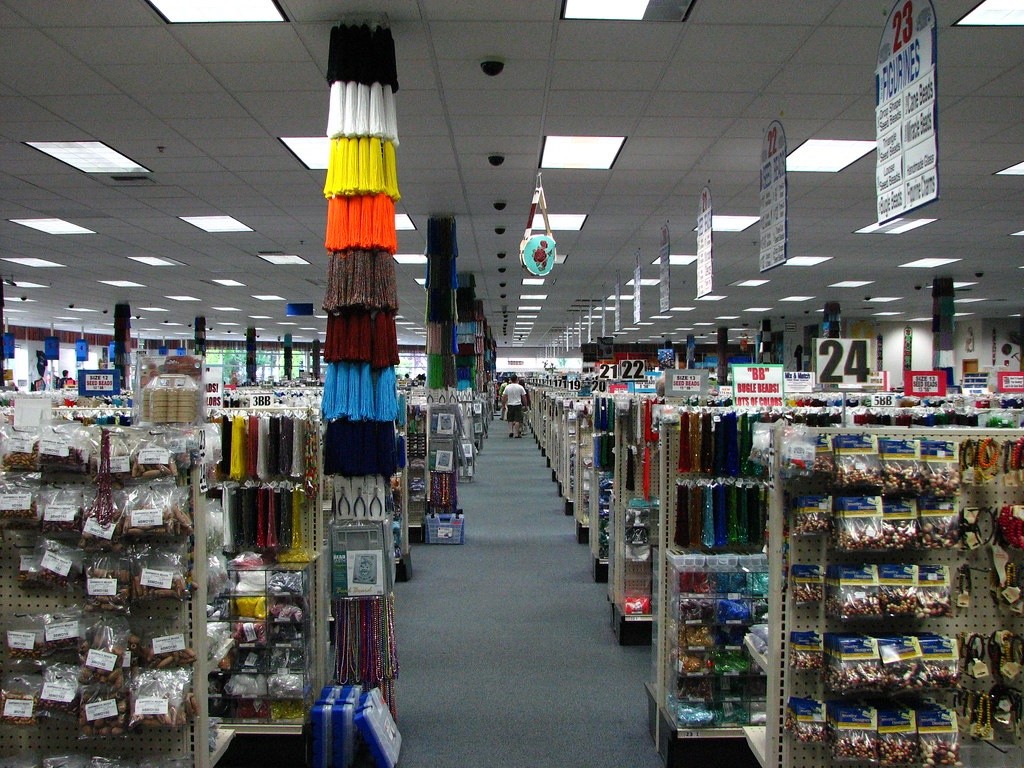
<box><xmin>674</xmin><ymin>408</ymin><xmax>978</xmax><ymax>548</ymax></box>
<box><xmin>96</xmin><ymin>429</ymin><xmax>114</xmax><ymax>530</ymax></box>
<box><xmin>430</xmin><ymin>470</ymin><xmax>459</xmax><ymax>514</ymax></box>
<box><xmin>333</xmin><ymin>594</ymin><xmax>400</xmax><ymax>727</ymax></box>
<box><xmin>304</xmin><ymin>408</ymin><xmax>320</xmax><ymax>498</ymax></box>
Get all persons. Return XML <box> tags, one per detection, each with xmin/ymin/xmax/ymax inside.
<box><xmin>59</xmin><ymin>370</ymin><xmax>75</xmax><ymax>389</ymax></box>
<box><xmin>495</xmin><ymin>375</ymin><xmax>532</xmax><ymax>438</ymax></box>
<box><xmin>404</xmin><ymin>373</ymin><xmax>426</xmax><ymax>383</ymax></box>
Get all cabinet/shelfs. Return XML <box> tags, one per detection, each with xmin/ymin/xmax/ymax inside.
<box><xmin>206</xmin><ymin>560</ymin><xmax>314</xmax><ymax>727</ymax></box>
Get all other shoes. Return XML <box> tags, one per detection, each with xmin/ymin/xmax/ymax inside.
<box><xmin>500</xmin><ymin>418</ymin><xmax>503</xmax><ymax>420</ymax></box>
<box><xmin>509</xmin><ymin>433</ymin><xmax>513</xmax><ymax>437</ymax></box>
<box><xmin>515</xmin><ymin>435</ymin><xmax>522</xmax><ymax>438</ymax></box>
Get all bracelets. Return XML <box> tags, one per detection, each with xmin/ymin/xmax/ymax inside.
<box><xmin>958</xmin><ymin>437</ymin><xmax>1024</xmax><ymax>739</ymax></box>
